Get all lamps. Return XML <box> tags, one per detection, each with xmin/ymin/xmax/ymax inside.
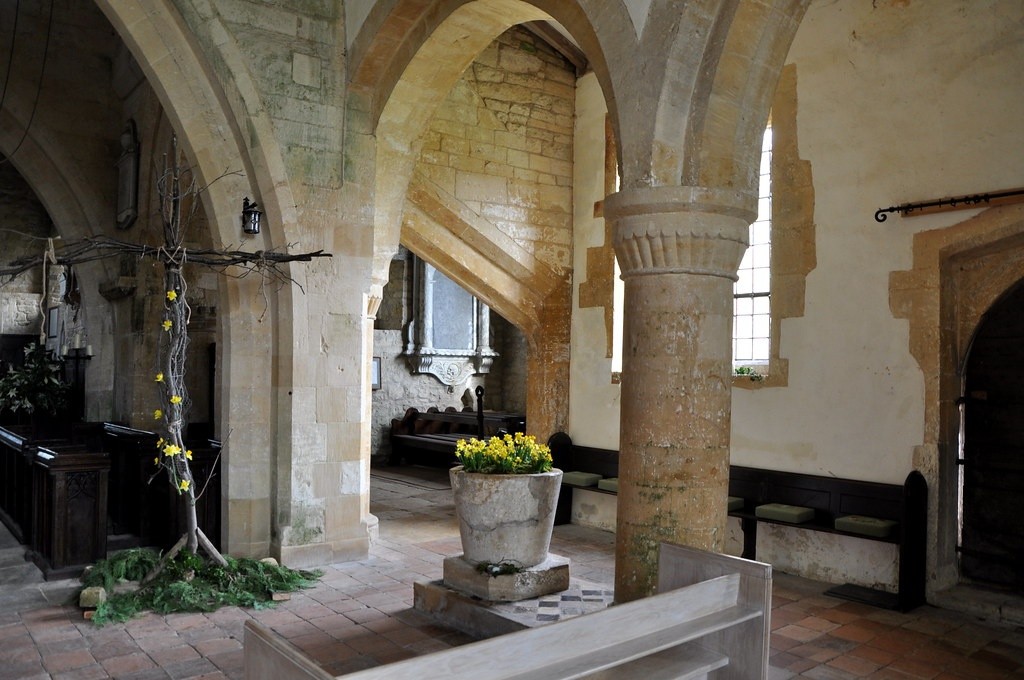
<box><xmin>242</xmin><ymin>197</ymin><xmax>261</xmax><ymax>234</ymax></box>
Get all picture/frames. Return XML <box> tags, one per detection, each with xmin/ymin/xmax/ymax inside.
<box><xmin>48</xmin><ymin>306</ymin><xmax>59</xmax><ymax>338</ymax></box>
<box><xmin>372</xmin><ymin>357</ymin><xmax>381</xmax><ymax>390</ymax></box>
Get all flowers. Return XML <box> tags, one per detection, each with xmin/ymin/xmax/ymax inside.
<box><xmin>0</xmin><ymin>342</ymin><xmax>71</xmax><ymax>414</ymax></box>
<box><xmin>153</xmin><ymin>289</ymin><xmax>194</xmax><ymax>493</ymax></box>
<box><xmin>455</xmin><ymin>432</ymin><xmax>553</xmax><ymax>474</ymax></box>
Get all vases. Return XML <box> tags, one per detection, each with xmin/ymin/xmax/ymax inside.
<box><xmin>449</xmin><ymin>465</ymin><xmax>563</xmax><ymax>569</ymax></box>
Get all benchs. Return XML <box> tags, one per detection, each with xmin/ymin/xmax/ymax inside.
<box><xmin>387</xmin><ymin>407</ymin><xmax>527</xmax><ymax>476</ymax></box>
<box><xmin>545</xmin><ymin>431</ymin><xmax>928</xmax><ymax>613</ymax></box>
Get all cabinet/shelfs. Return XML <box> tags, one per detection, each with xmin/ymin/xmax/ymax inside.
<box><xmin>242</xmin><ymin>539</ymin><xmax>773</xmax><ymax>680</ymax></box>
